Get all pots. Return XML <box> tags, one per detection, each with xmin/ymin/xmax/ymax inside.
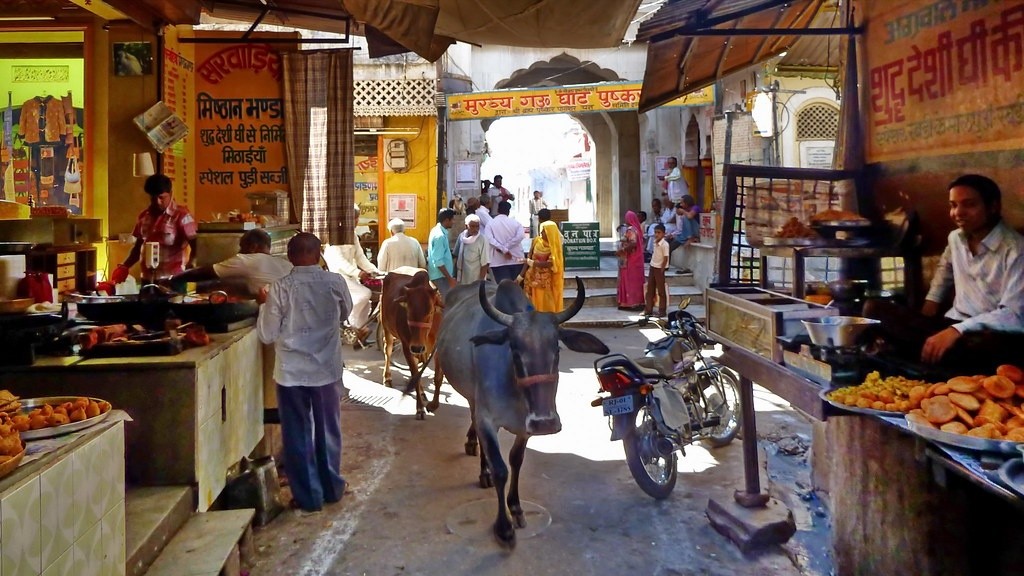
<box><xmin>167</xmin><ymin>287</ymin><xmax>261</xmax><ymax>327</ymax></box>
<box><xmin>78</xmin><ymin>286</ymin><xmax>175</xmax><ymax>323</ymax></box>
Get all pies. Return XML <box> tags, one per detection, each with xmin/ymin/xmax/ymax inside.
<box><xmin>836</xmin><ymin>363</ymin><xmax>1024</xmax><ymax>444</ymax></box>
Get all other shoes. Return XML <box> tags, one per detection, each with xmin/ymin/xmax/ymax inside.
<box><xmin>676</xmin><ymin>269</ymin><xmax>689</xmax><ymax>274</ymax></box>
<box><xmin>353</xmin><ymin>340</ymin><xmax>375</xmax><ymax>349</ymax></box>
<box><xmin>618</xmin><ymin>306</ymin><xmax>631</xmax><ymax>310</ymax></box>
<box><xmin>654</xmin><ymin>313</ymin><xmax>666</xmax><ymax>317</ymax></box>
<box><xmin>633</xmin><ymin>305</ymin><xmax>645</xmax><ymax>311</ymax></box>
<box><xmin>639</xmin><ymin>310</ymin><xmax>653</xmax><ymax>316</ymax></box>
<box><xmin>355</xmin><ymin>326</ymin><xmax>372</xmax><ymax>345</ymax></box>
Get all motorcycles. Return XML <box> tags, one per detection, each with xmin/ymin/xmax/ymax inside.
<box><xmin>592</xmin><ymin>296</ymin><xmax>741</xmax><ymax>501</ymax></box>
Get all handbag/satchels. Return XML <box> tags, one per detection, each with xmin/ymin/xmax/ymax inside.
<box><xmin>234</xmin><ymin>455</ymin><xmax>284</xmax><ymax>527</ymax></box>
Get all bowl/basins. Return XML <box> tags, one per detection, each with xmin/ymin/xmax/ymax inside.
<box><xmin>801</xmin><ymin>315</ymin><xmax>881</xmax><ymax>347</ymax></box>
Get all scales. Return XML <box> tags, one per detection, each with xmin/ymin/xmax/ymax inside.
<box><xmin>775</xmin><ymin>316</ymin><xmax>881</xmax><ymax>388</ymax></box>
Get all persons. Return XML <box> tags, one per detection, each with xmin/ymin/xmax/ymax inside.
<box><xmin>256</xmin><ymin>233</ymin><xmax>353</xmax><ymax>512</ymax></box>
<box><xmin>324</xmin><ymin>204</ymin><xmax>385</xmax><ymax>344</ymax></box>
<box><xmin>123</xmin><ymin>174</ymin><xmax>198</xmax><ymax>294</ymax></box>
<box><xmin>617</xmin><ymin>157</ymin><xmax>704</xmax><ymax>264</ymax></box>
<box><xmin>450</xmin><ymin>176</ymin><xmax>525</xmax><ymax>287</ymax></box>
<box><xmin>615</xmin><ymin>210</ymin><xmax>645</xmax><ymax>318</ymax></box>
<box><xmin>365</xmin><ymin>208</ymin><xmax>454</xmax><ymax>282</ymax></box>
<box><xmin>142</xmin><ymin>228</ymin><xmax>293</xmax><ymax>300</ymax></box>
<box><xmin>528</xmin><ymin>191</ymin><xmax>548</xmax><ymax>238</ymax></box>
<box><xmin>514</xmin><ymin>208</ymin><xmax>565</xmax><ymax>313</ymax></box>
<box><xmin>639</xmin><ymin>224</ymin><xmax>669</xmax><ymax>322</ymax></box>
<box><xmin>920</xmin><ymin>174</ymin><xmax>1024</xmax><ymax>364</ymax></box>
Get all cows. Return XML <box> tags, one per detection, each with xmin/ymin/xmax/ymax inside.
<box><xmin>379</xmin><ymin>269</ymin><xmax>444</xmax><ymax>421</ymax></box>
<box><xmin>402</xmin><ymin>274</ymin><xmax>611</xmax><ymax>551</ymax></box>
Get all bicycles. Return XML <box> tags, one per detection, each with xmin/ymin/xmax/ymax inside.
<box><xmin>339</xmin><ymin>275</ymin><xmax>433</xmax><ymax>372</ymax></box>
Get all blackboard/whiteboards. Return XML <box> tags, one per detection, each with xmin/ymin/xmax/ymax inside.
<box><xmin>561</xmin><ymin>222</ymin><xmax>600</xmax><ymax>266</ymax></box>
